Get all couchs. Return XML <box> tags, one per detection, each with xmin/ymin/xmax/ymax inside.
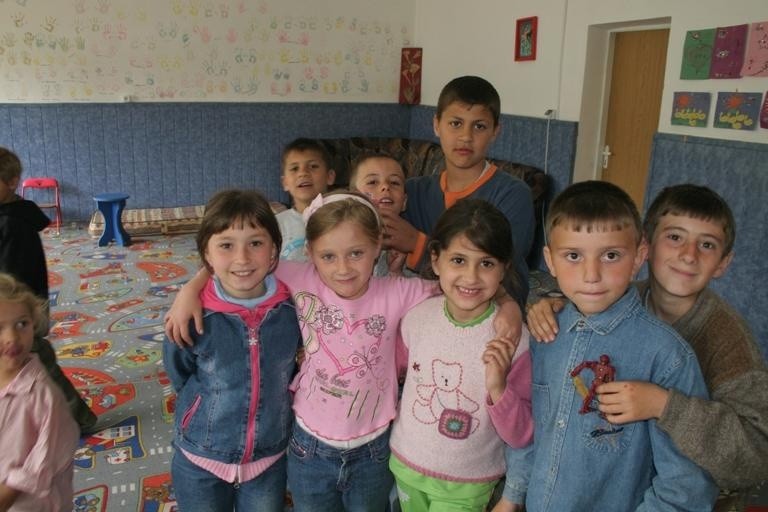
<box><xmin>301</xmin><ymin>137</ymin><xmax>551</xmax><ymax>265</ymax></box>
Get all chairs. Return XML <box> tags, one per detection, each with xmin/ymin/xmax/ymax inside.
<box><xmin>23</xmin><ymin>178</ymin><xmax>63</xmax><ymax>232</ymax></box>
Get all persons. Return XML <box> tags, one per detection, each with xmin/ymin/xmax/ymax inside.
<box><xmin>491</xmin><ymin>181</ymin><xmax>720</xmax><ymax>510</ymax></box>
<box><xmin>275</xmin><ymin>76</ymin><xmax>536</xmax><ymax>309</ymax></box>
<box><xmin>526</xmin><ymin>184</ymin><xmax>768</xmax><ymax>492</ymax></box>
<box><xmin>164</xmin><ymin>188</ymin><xmax>522</xmax><ymax>512</ymax></box>
<box><xmin>0</xmin><ymin>274</ymin><xmax>79</xmax><ymax>511</ymax></box>
<box><xmin>0</xmin><ymin>146</ymin><xmax>96</xmax><ymax>431</ymax></box>
<box><xmin>162</xmin><ymin>189</ymin><xmax>301</xmax><ymax>511</ymax></box>
<box><xmin>297</xmin><ymin>198</ymin><xmax>535</xmax><ymax>511</ymax></box>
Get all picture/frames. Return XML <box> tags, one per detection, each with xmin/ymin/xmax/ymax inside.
<box><xmin>515</xmin><ymin>16</ymin><xmax>538</xmax><ymax>63</ymax></box>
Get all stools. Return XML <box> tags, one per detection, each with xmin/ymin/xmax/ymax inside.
<box><xmin>93</xmin><ymin>191</ymin><xmax>131</xmax><ymax>246</ymax></box>
